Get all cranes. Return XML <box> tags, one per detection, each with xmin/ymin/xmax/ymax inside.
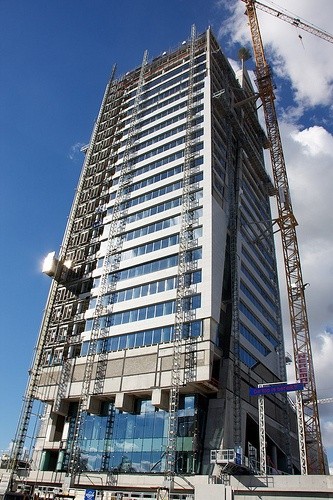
<box><xmin>242</xmin><ymin>0</ymin><xmax>333</xmax><ymax>474</ymax></box>
